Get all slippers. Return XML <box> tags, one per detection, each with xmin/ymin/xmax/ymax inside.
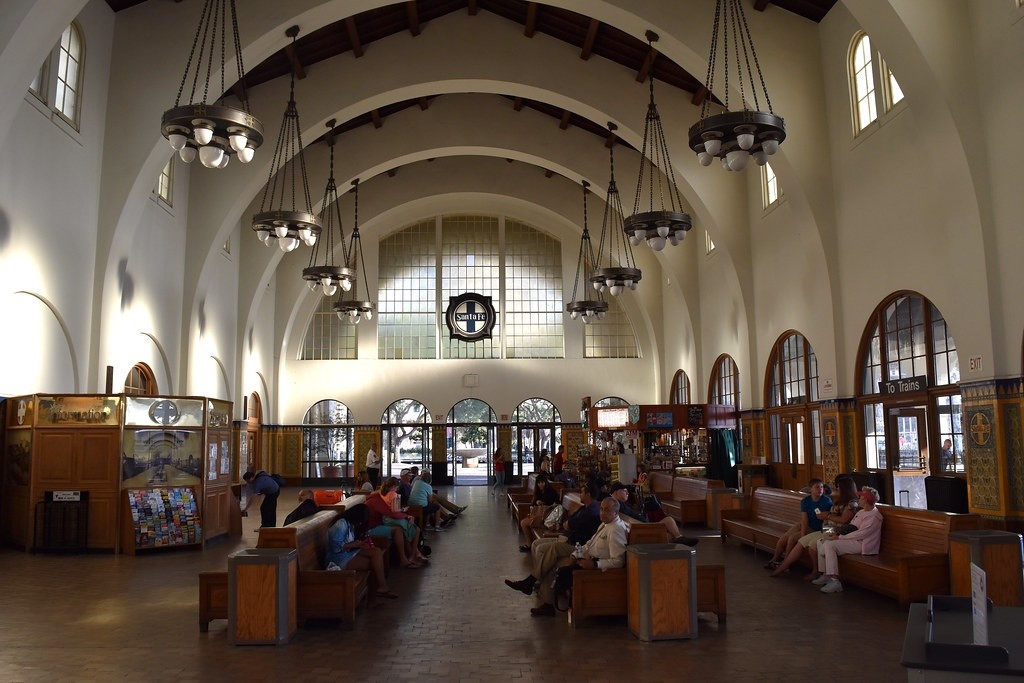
<box><xmin>408</xmin><ymin>558</ymin><xmax>425</xmax><ymax>566</ymax></box>
<box><xmin>416</xmin><ymin>553</ymin><xmax>432</xmax><ymax>561</ymax></box>
<box><xmin>399</xmin><ymin>562</ymin><xmax>417</xmax><ymax>569</ymax></box>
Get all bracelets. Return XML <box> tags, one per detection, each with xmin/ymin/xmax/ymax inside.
<box><xmin>827</xmin><ymin>512</ymin><xmax>831</xmax><ymax>519</ymax></box>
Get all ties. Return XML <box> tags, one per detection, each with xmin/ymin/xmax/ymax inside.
<box><xmin>589</xmin><ymin>525</ymin><xmax>605</xmax><ymax>546</ymax></box>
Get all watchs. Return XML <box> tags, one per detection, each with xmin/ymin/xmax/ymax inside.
<box><xmin>592</xmin><ymin>560</ymin><xmax>597</xmax><ymax>570</ymax></box>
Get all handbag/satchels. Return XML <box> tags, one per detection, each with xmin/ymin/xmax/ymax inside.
<box><xmin>834</xmin><ymin>523</ymin><xmax>858</xmax><ymax>535</ymax></box>
<box><xmin>529</xmin><ymin>504</ymin><xmax>551</xmax><ymax>527</ymax></box>
<box><xmin>271</xmin><ymin>473</ymin><xmax>287</xmax><ymax>487</ymax></box>
<box><xmin>552</xmin><ymin>565</ymin><xmax>573</xmax><ymax>612</ymax></box>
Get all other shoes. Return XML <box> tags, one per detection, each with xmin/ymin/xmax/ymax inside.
<box><xmin>491</xmin><ymin>488</ymin><xmax>506</xmax><ymax>496</ymax></box>
<box><xmin>519</xmin><ymin>544</ymin><xmax>530</xmax><ymax>551</ymax></box>
<box><xmin>253</xmin><ymin>528</ymin><xmax>259</xmax><ymax>532</ymax></box>
<box><xmin>763</xmin><ymin>558</ymin><xmax>822</xmax><ymax>581</ymax></box>
<box><xmin>440</xmin><ymin>504</ymin><xmax>468</xmax><ymax>527</ymax></box>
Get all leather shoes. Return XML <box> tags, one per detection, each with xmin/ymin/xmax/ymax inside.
<box><xmin>504</xmin><ymin>578</ymin><xmax>532</xmax><ymax>595</ymax></box>
<box><xmin>530</xmin><ymin>603</ymin><xmax>555</xmax><ymax>617</ymax></box>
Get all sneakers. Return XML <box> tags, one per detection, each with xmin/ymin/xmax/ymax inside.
<box><xmin>435</xmin><ymin>526</ymin><xmax>447</xmax><ymax>531</ymax></box>
<box><xmin>820</xmin><ymin>578</ymin><xmax>843</xmax><ymax>593</ymax></box>
<box><xmin>375</xmin><ymin>589</ymin><xmax>399</xmax><ymax>598</ymax></box>
<box><xmin>672</xmin><ymin>535</ymin><xmax>699</xmax><ymax>547</ymax></box>
<box><xmin>811</xmin><ymin>574</ymin><xmax>832</xmax><ymax>585</ymax></box>
<box><xmin>424</xmin><ymin>525</ymin><xmax>434</xmax><ymax>530</ymax></box>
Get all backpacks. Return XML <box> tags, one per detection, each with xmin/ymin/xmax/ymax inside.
<box><xmin>543</xmin><ymin>504</ymin><xmax>566</xmax><ymax>530</ymax></box>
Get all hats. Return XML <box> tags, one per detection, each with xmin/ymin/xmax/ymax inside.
<box><xmin>400</xmin><ymin>468</ymin><xmax>430</xmax><ymax>476</ymax></box>
<box><xmin>857</xmin><ymin>488</ymin><xmax>877</xmax><ymax>504</ymax></box>
<box><xmin>611</xmin><ymin>481</ymin><xmax>625</xmax><ymax>494</ymax></box>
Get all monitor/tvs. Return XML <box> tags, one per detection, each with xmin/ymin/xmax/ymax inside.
<box><xmin>598</xmin><ymin>408</ymin><xmax>629</xmax><ymax>427</ymax></box>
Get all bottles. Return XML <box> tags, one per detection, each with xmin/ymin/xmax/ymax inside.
<box><xmin>575</xmin><ymin>541</ymin><xmax>583</xmax><ymax>558</ymax></box>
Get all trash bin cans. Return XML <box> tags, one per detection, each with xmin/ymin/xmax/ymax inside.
<box><xmin>626</xmin><ymin>542</ymin><xmax>700</xmax><ymax>642</ymax></box>
<box><xmin>560</xmin><ymin>487</ymin><xmax>577</xmax><ymax>503</ymax></box>
<box><xmin>947</xmin><ymin>528</ymin><xmax>1024</xmax><ymax>608</ymax></box>
<box><xmin>226</xmin><ymin>546</ymin><xmax>299</xmax><ymax>647</ymax></box>
<box><xmin>706</xmin><ymin>488</ymin><xmax>732</xmax><ymax>530</ymax></box>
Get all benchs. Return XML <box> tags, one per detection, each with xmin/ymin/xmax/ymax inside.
<box><xmin>178</xmin><ymin>463</ymin><xmax>1024</xmax><ymax>648</ymax></box>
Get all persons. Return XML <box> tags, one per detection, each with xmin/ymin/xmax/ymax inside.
<box><xmin>241</xmin><ymin>470</ymin><xmax>280</xmax><ymax>532</ymax></box>
<box><xmin>765</xmin><ymin>478</ymin><xmax>833</xmax><ymax>574</ymax></box>
<box><xmin>490</xmin><ymin>440</ymin><xmax>568</xmax><ymax>497</ymax></box>
<box><xmin>365</xmin><ymin>442</ymin><xmax>383</xmax><ymax>492</ymax></box>
<box><xmin>617</xmin><ymin>442</ymin><xmax>625</xmax><ymax>454</ymax></box>
<box><xmin>941</xmin><ymin>439</ymin><xmax>954</xmax><ymax>470</ymax></box>
<box><xmin>322</xmin><ymin>466</ymin><xmax>468</xmax><ymax>602</ymax></box>
<box><xmin>608</xmin><ymin>473</ymin><xmax>700</xmax><ymax>547</ymax></box>
<box><xmin>518</xmin><ymin>474</ymin><xmax>560</xmax><ymax>552</ymax></box>
<box><xmin>528</xmin><ymin>482</ymin><xmax>602</xmax><ymax>573</ymax></box>
<box><xmin>811</xmin><ymin>486</ymin><xmax>884</xmax><ymax>592</ymax></box>
<box><xmin>283</xmin><ymin>489</ymin><xmax>319</xmax><ymax>526</ymax></box>
<box><xmin>505</xmin><ymin>498</ymin><xmax>629</xmax><ymax>617</ymax></box>
<box><xmin>769</xmin><ymin>476</ymin><xmax>861</xmax><ymax>581</ymax></box>
<box><xmin>593</xmin><ymin>478</ymin><xmax>612</xmax><ymax>502</ymax></box>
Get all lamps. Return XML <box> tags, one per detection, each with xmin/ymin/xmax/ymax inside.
<box><xmin>620</xmin><ymin>29</ymin><xmax>692</xmax><ymax>250</ymax></box>
<box><xmin>248</xmin><ymin>24</ymin><xmax>324</xmax><ymax>252</ymax></box>
<box><xmin>563</xmin><ymin>177</ymin><xmax>610</xmax><ymax>323</ymax></box>
<box><xmin>330</xmin><ymin>175</ymin><xmax>377</xmax><ymax>324</ymax></box>
<box><xmin>156</xmin><ymin>0</ymin><xmax>265</xmax><ymax>171</ymax></box>
<box><xmin>688</xmin><ymin>0</ymin><xmax>787</xmax><ymax>171</ymax></box>
<box><xmin>300</xmin><ymin>114</ymin><xmax>356</xmax><ymax>296</ymax></box>
<box><xmin>588</xmin><ymin>121</ymin><xmax>642</xmax><ymax>297</ymax></box>
